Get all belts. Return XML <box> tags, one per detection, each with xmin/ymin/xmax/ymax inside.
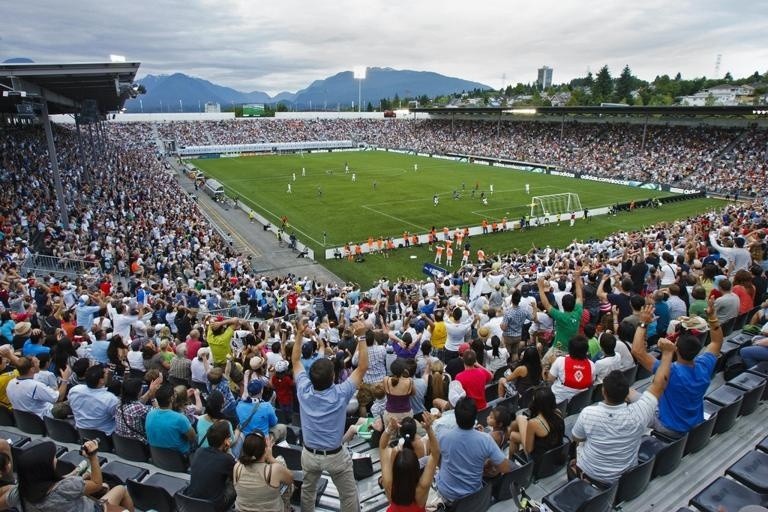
<box><xmin>302</xmin><ymin>444</ymin><xmax>342</xmax><ymax>455</ymax></box>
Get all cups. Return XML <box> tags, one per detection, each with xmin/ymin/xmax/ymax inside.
<box><xmin>430</xmin><ymin>407</ymin><xmax>441</xmax><ymax>419</ymax></box>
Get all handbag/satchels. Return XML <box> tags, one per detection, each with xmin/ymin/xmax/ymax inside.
<box><xmin>511</xmin><ymin>480</ymin><xmax>546</xmax><ymax>512</ymax></box>
<box><xmin>368</xmin><ymin>431</ymin><xmax>383</xmax><ymax>447</ymax></box>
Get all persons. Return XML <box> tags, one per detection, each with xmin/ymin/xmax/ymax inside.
<box><xmin>1</xmin><ymin>120</ymin><xmax>767</xmax><ymax>512</ymax></box>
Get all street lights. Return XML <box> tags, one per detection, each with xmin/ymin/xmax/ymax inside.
<box><xmin>353</xmin><ymin>65</ymin><xmax>368</xmax><ymax>113</ymax></box>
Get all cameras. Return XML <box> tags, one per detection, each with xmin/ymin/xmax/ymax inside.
<box><xmin>79</xmin><ymin>438</ymin><xmax>101</xmax><ymax>456</ymax></box>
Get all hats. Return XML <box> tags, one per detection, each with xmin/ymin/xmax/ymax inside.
<box><xmin>43</xmin><ymin>276</ymin><xmax>51</xmax><ymax>280</ymax></box>
<box><xmin>476</xmin><ymin>326</ymin><xmax>491</xmax><ymax>341</ymax></box>
<box><xmin>274</xmin><ymin>360</ymin><xmax>290</xmax><ymax>372</ymax></box>
<box><xmin>197</xmin><ymin>345</ymin><xmax>212</xmax><ymax>357</ymax></box>
<box><xmin>155</xmin><ymin>323</ymin><xmax>165</xmax><ymax>332</ymax></box>
<box><xmin>249</xmin><ymin>355</ymin><xmax>265</xmax><ymax>370</ymax></box>
<box><xmin>28</xmin><ymin>280</ymin><xmax>35</xmax><ymax>288</ymax></box>
<box><xmin>54</xmin><ymin>296</ymin><xmax>60</xmax><ymax>302</ymax></box>
<box><xmin>8</xmin><ymin>292</ymin><xmax>17</xmax><ymax>298</ymax></box>
<box><xmin>14</xmin><ymin>322</ymin><xmax>33</xmax><ymax>336</ymax></box>
<box><xmin>78</xmin><ymin>294</ymin><xmax>89</xmax><ymax>306</ymax></box>
<box><xmin>247</xmin><ymin>379</ymin><xmax>265</xmax><ymax>396</ymax></box>
